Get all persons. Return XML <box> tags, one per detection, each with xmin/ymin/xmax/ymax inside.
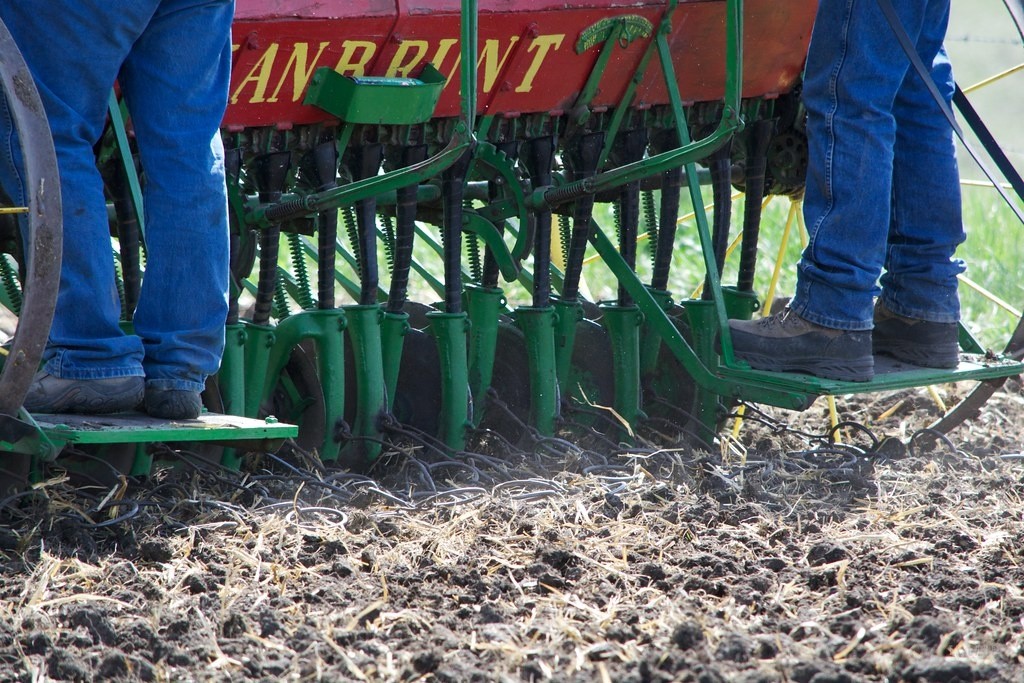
<box><xmin>713</xmin><ymin>0</ymin><xmax>968</xmax><ymax>381</ymax></box>
<box><xmin>0</xmin><ymin>0</ymin><xmax>235</xmax><ymax>421</ymax></box>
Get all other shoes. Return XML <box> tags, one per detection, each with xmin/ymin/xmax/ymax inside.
<box><xmin>147</xmin><ymin>389</ymin><xmax>205</xmax><ymax>419</ymax></box>
<box><xmin>877</xmin><ymin>302</ymin><xmax>959</xmax><ymax>371</ymax></box>
<box><xmin>716</xmin><ymin>306</ymin><xmax>873</xmax><ymax>383</ymax></box>
<box><xmin>20</xmin><ymin>366</ymin><xmax>145</xmax><ymax>416</ymax></box>
